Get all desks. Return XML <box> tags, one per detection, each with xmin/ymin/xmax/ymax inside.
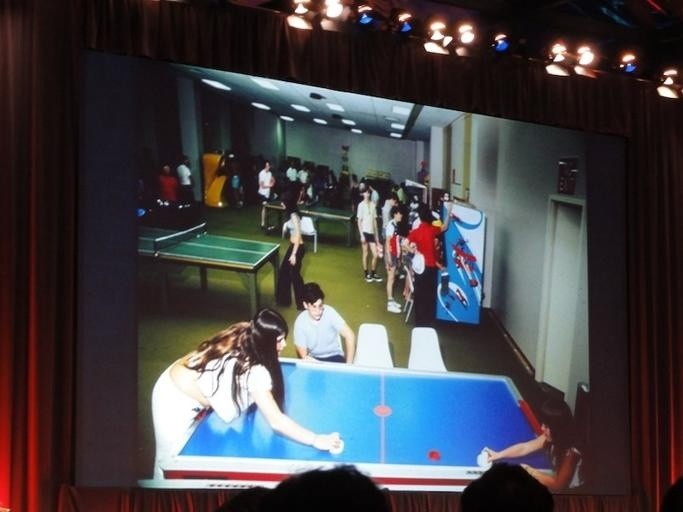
<box><xmin>261</xmin><ymin>198</ymin><xmax>356</xmax><ymax>248</ymax></box>
<box><xmin>136</xmin><ymin>218</ymin><xmax>280</xmax><ymax>319</ymax></box>
<box><xmin>155</xmin><ymin>354</ymin><xmax>554</xmax><ymax>484</ymax></box>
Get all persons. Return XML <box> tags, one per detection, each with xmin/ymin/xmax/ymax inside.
<box><xmin>355</xmin><ymin>173</ymin><xmax>457</xmax><ymax>325</ymax></box>
<box><xmin>473</xmin><ymin>392</ymin><xmax>629</xmax><ymax>493</ymax></box>
<box><xmin>272</xmin><ymin>463</ymin><xmax>395</xmax><ymax>512</ymax></box>
<box><xmin>144</xmin><ymin>309</ymin><xmax>346</xmax><ymax>478</ymax></box>
<box><xmin>296</xmin><ymin>283</ymin><xmax>357</xmax><ymax>365</ymax></box>
<box><xmin>265</xmin><ymin>193</ymin><xmax>309</xmax><ymax>312</ymax></box>
<box><xmin>453</xmin><ymin>464</ymin><xmax>559</xmax><ymax>512</ymax></box>
<box><xmin>214</xmin><ymin>485</ymin><xmax>274</xmax><ymax>510</ymax></box>
<box><xmin>137</xmin><ymin>146</ymin><xmax>361</xmax><ymax>228</ymax></box>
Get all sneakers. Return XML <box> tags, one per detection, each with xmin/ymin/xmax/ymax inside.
<box><xmin>365</xmin><ymin>273</ymin><xmax>373</xmax><ymax>282</ymax></box>
<box><xmin>372</xmin><ymin>273</ymin><xmax>384</xmax><ymax>282</ymax></box>
<box><xmin>387</xmin><ymin>305</ymin><xmax>402</xmax><ymax>313</ymax></box>
<box><xmin>395</xmin><ymin>302</ymin><xmax>402</xmax><ymax>308</ymax></box>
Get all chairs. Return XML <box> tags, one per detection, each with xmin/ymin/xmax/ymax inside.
<box><xmin>351</xmin><ymin>323</ymin><xmax>448</xmax><ymax>373</ymax></box>
